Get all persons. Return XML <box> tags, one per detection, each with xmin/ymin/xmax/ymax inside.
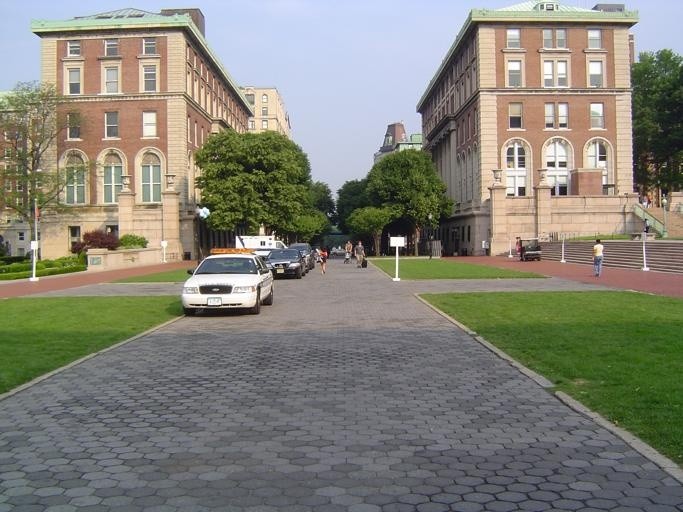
<box><xmin>592</xmin><ymin>239</ymin><xmax>603</xmax><ymax>277</ymax></box>
<box><xmin>642</xmin><ymin>193</ymin><xmax>648</xmax><ymax>208</ymax></box>
<box><xmin>647</xmin><ymin>194</ymin><xmax>651</xmax><ymax>208</ymax></box>
<box><xmin>354</xmin><ymin>240</ymin><xmax>365</xmax><ymax>268</ymax></box>
<box><xmin>318</xmin><ymin>247</ymin><xmax>327</xmax><ymax>275</ymax></box>
<box><xmin>331</xmin><ymin>245</ymin><xmax>336</xmax><ymax>259</ymax></box>
<box><xmin>644</xmin><ymin>219</ymin><xmax>649</xmax><ymax>233</ymax></box>
<box><xmin>345</xmin><ymin>240</ymin><xmax>352</xmax><ymax>263</ymax></box>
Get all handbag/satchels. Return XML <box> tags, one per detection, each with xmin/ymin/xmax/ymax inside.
<box><xmin>316</xmin><ymin>256</ymin><xmax>323</xmax><ymax>264</ymax></box>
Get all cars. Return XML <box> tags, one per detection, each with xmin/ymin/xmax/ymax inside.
<box><xmin>180</xmin><ymin>236</ymin><xmax>321</xmax><ymax>316</ymax></box>
<box><xmin>330</xmin><ymin>248</ymin><xmax>346</xmax><ymax>257</ymax></box>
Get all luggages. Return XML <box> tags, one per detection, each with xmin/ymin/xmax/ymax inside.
<box><xmin>362</xmin><ymin>255</ymin><xmax>367</xmax><ymax>268</ymax></box>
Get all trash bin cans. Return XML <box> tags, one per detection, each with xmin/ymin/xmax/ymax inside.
<box><xmin>461</xmin><ymin>248</ymin><xmax>467</xmax><ymax>256</ymax></box>
<box><xmin>184</xmin><ymin>252</ymin><xmax>191</xmax><ymax>260</ymax></box>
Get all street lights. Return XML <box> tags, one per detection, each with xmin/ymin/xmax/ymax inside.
<box><xmin>427</xmin><ymin>213</ymin><xmax>433</xmax><ymax>258</ymax></box>
<box><xmin>30</xmin><ymin>169</ymin><xmax>42</xmax><ymax>281</ymax></box>
<box><xmin>661</xmin><ymin>198</ymin><xmax>668</xmax><ymax>238</ymax></box>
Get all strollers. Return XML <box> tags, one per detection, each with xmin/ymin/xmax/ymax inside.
<box><xmin>343</xmin><ymin>251</ymin><xmax>351</xmax><ymax>265</ymax></box>
